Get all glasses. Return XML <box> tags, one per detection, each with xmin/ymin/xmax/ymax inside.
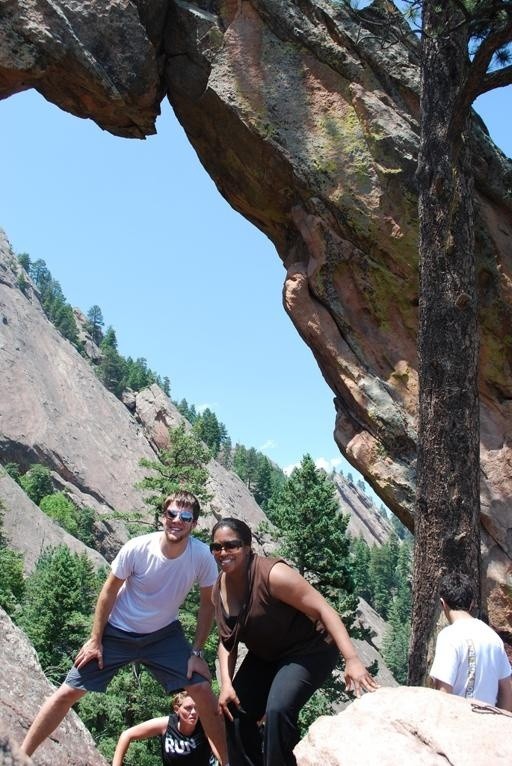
<box><xmin>165</xmin><ymin>509</ymin><xmax>193</xmax><ymax>522</ymax></box>
<box><xmin>209</xmin><ymin>540</ymin><xmax>242</xmax><ymax>554</ymax></box>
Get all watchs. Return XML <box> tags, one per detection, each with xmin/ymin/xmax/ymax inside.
<box><xmin>190</xmin><ymin>649</ymin><xmax>205</xmax><ymax>659</ymax></box>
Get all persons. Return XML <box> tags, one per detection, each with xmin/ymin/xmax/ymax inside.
<box><xmin>14</xmin><ymin>489</ymin><xmax>234</xmax><ymax>766</ymax></box>
<box><xmin>110</xmin><ymin>691</ymin><xmax>220</xmax><ymax>766</ymax></box>
<box><xmin>209</xmin><ymin>517</ymin><xmax>382</xmax><ymax>765</ymax></box>
<box><xmin>426</xmin><ymin>570</ymin><xmax>511</xmax><ymax>714</ymax></box>
